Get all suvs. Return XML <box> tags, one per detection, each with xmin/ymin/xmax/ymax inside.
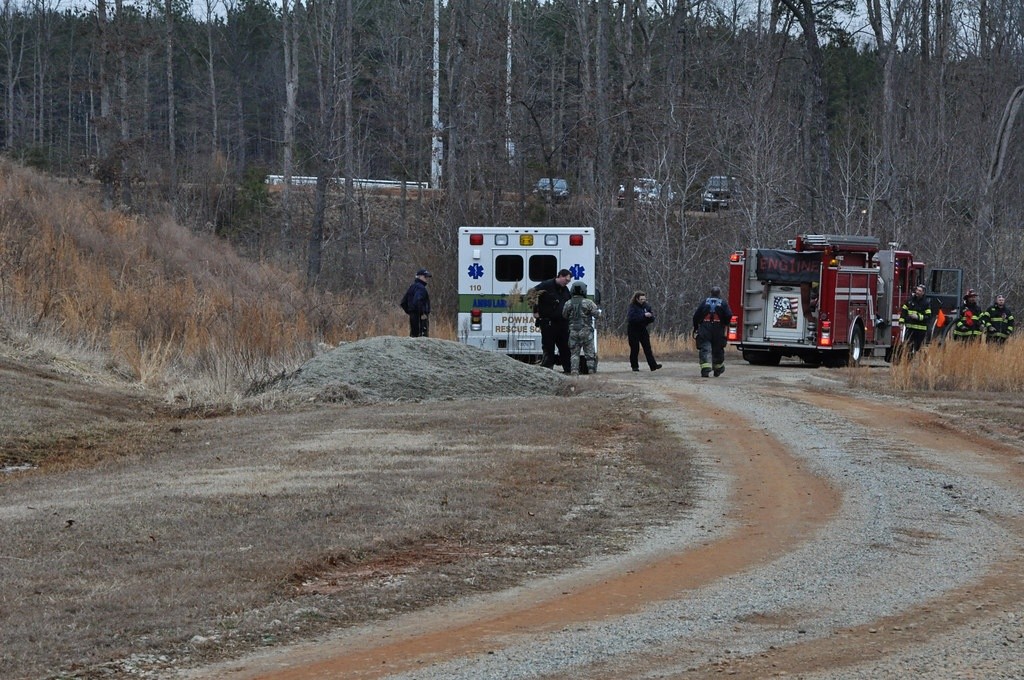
<box><xmin>699</xmin><ymin>175</ymin><xmax>746</xmax><ymax>212</ymax></box>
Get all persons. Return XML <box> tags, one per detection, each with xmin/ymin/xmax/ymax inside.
<box><xmin>627</xmin><ymin>290</ymin><xmax>662</xmax><ymax>372</ymax></box>
<box><xmin>401</xmin><ymin>269</ymin><xmax>432</xmax><ymax>338</ymax></box>
<box><xmin>526</xmin><ymin>269</ymin><xmax>573</xmax><ymax>375</ymax></box>
<box><xmin>983</xmin><ymin>294</ymin><xmax>1014</xmax><ymax>352</ymax></box>
<box><xmin>899</xmin><ymin>285</ymin><xmax>931</xmax><ymax>363</ymax></box>
<box><xmin>562</xmin><ymin>281</ymin><xmax>602</xmax><ymax>376</ymax></box>
<box><xmin>692</xmin><ymin>286</ymin><xmax>732</xmax><ymax>378</ymax></box>
<box><xmin>954</xmin><ymin>289</ymin><xmax>983</xmax><ymax>350</ymax></box>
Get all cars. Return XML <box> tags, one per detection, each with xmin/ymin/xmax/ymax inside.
<box><xmin>617</xmin><ymin>177</ymin><xmax>677</xmax><ymax>210</ymax></box>
<box><xmin>533</xmin><ymin>178</ymin><xmax>571</xmax><ymax>203</ymax></box>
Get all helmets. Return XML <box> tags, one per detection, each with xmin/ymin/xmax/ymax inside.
<box><xmin>570</xmin><ymin>281</ymin><xmax>587</xmax><ymax>298</ymax></box>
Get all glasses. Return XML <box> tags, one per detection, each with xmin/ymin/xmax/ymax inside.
<box><xmin>998</xmin><ymin>295</ymin><xmax>1005</xmax><ymax>298</ymax></box>
<box><xmin>565</xmin><ymin>276</ymin><xmax>570</xmax><ymax>282</ymax></box>
<box><xmin>919</xmin><ymin>284</ymin><xmax>926</xmax><ymax>290</ymax></box>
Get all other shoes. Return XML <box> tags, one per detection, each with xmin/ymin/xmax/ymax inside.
<box><xmin>701</xmin><ymin>367</ymin><xmax>712</xmax><ymax>378</ymax></box>
<box><xmin>713</xmin><ymin>366</ymin><xmax>725</xmax><ymax>377</ymax></box>
<box><xmin>632</xmin><ymin>365</ymin><xmax>639</xmax><ymax>371</ymax></box>
<box><xmin>649</xmin><ymin>364</ymin><xmax>663</xmax><ymax>372</ymax></box>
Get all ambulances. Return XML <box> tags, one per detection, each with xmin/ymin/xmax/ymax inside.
<box><xmin>456</xmin><ymin>221</ymin><xmax>598</xmax><ymax>372</ymax></box>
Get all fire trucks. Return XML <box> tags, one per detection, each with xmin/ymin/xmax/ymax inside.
<box><xmin>722</xmin><ymin>234</ymin><xmax>969</xmax><ymax>371</ymax></box>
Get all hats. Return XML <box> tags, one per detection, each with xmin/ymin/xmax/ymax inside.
<box><xmin>417</xmin><ymin>270</ymin><xmax>432</xmax><ymax>277</ymax></box>
<box><xmin>962</xmin><ymin>288</ymin><xmax>978</xmax><ymax>300</ymax></box>
